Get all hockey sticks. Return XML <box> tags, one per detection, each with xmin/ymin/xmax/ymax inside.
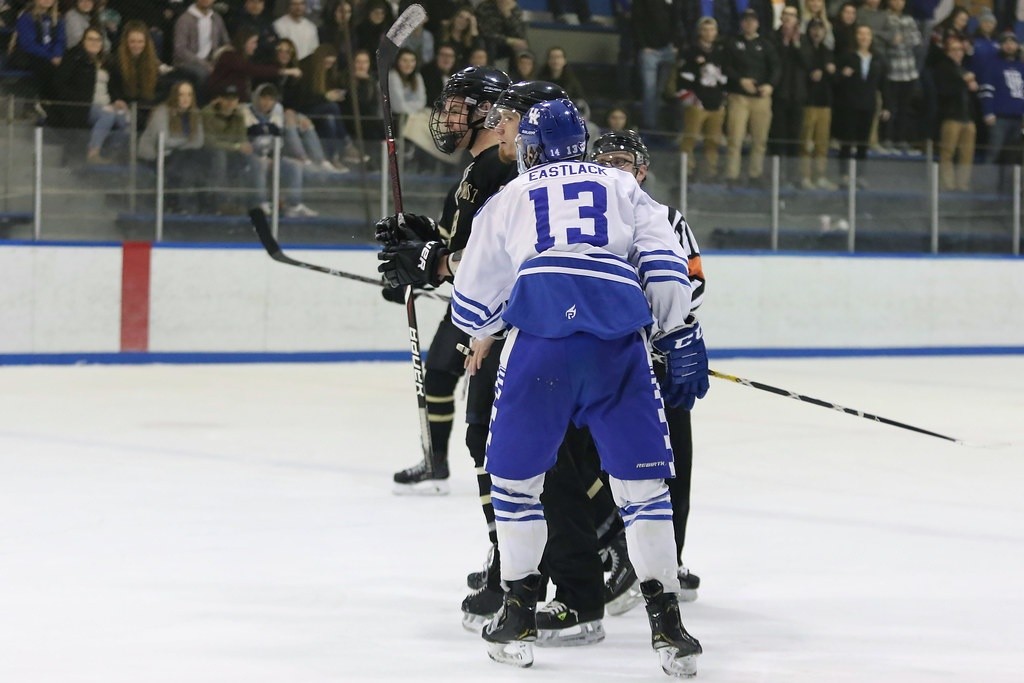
<box><xmin>650</xmin><ymin>351</ymin><xmax>963</xmax><ymax>445</ymax></box>
<box><xmin>247</xmin><ymin>204</ymin><xmax>453</xmax><ymax>304</ymax></box>
<box><xmin>371</xmin><ymin>1</ymin><xmax>437</xmax><ymax>476</ymax></box>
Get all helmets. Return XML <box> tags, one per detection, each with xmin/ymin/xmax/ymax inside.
<box><xmin>518</xmin><ymin>98</ymin><xmax>589</xmax><ymax>164</ymax></box>
<box><xmin>429</xmin><ymin>65</ymin><xmax>515</xmax><ymax>155</ymax></box>
<box><xmin>494</xmin><ymin>79</ymin><xmax>568</xmax><ymax>114</ymax></box>
<box><xmin>592</xmin><ymin>129</ymin><xmax>651</xmax><ymax>169</ymax></box>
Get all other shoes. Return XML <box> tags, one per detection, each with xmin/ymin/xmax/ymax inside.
<box><xmin>727</xmin><ymin>177</ymin><xmax>771</xmax><ymax>191</ymax></box>
<box><xmin>331</xmin><ymin>154</ymin><xmax>349</xmax><ymax>173</ymax></box>
<box><xmin>287</xmin><ymin>205</ymin><xmax>319</xmax><ymax>219</ymax></box>
<box><xmin>343</xmin><ymin>143</ymin><xmax>370</xmax><ymax>163</ymax></box>
<box><xmin>86</xmin><ymin>153</ymin><xmax>111</xmax><ymax>166</ymax></box>
<box><xmin>800</xmin><ymin>175</ymin><xmax>840</xmax><ymax>192</ymax></box>
<box><xmin>260</xmin><ymin>202</ymin><xmax>280</xmax><ymax>218</ymax></box>
<box><xmin>299</xmin><ymin>159</ymin><xmax>339</xmax><ymax>175</ymax></box>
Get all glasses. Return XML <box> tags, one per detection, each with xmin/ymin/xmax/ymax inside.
<box><xmin>595</xmin><ymin>157</ymin><xmax>635</xmax><ymax>168</ymax></box>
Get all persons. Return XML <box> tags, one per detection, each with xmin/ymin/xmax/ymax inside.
<box><xmin>619</xmin><ymin>0</ymin><xmax>1024</xmax><ymax>197</ymax></box>
<box><xmin>449</xmin><ymin>98</ymin><xmax>706</xmax><ymax>678</ymax></box>
<box><xmin>1</xmin><ymin>0</ymin><xmax>645</xmax><ymax>223</ymax></box>
<box><xmin>376</xmin><ymin>67</ymin><xmax>704</xmax><ymax>645</ymax></box>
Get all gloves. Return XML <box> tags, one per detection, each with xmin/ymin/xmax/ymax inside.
<box><xmin>382</xmin><ymin>284</ymin><xmax>437</xmax><ymax>304</ymax></box>
<box><xmin>651</xmin><ymin>313</ymin><xmax>709</xmax><ymax>412</ymax></box>
<box><xmin>378</xmin><ymin>240</ymin><xmax>451</xmax><ymax>288</ymax></box>
<box><xmin>374</xmin><ymin>212</ymin><xmax>441</xmax><ymax>242</ymax></box>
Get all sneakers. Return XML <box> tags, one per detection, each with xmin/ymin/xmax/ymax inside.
<box><xmin>466</xmin><ymin>547</ymin><xmax>498</xmax><ymax>589</ymax></box>
<box><xmin>391</xmin><ymin>453</ymin><xmax>450</xmax><ymax>495</ymax></box>
<box><xmin>536</xmin><ymin>595</ymin><xmax>607</xmax><ymax>648</ymax></box>
<box><xmin>677</xmin><ymin>567</ymin><xmax>700</xmax><ymax>601</ymax></box>
<box><xmin>461</xmin><ymin>584</ymin><xmax>505</xmax><ymax>633</ymax></box>
<box><xmin>639</xmin><ymin>578</ymin><xmax>703</xmax><ymax>678</ymax></box>
<box><xmin>599</xmin><ymin>532</ymin><xmax>643</xmax><ymax>616</ymax></box>
<box><xmin>481</xmin><ymin>592</ymin><xmax>537</xmax><ymax>669</ymax></box>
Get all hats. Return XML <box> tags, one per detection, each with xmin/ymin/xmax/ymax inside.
<box><xmin>220</xmin><ymin>83</ymin><xmax>241</xmax><ymax>101</ymax></box>
